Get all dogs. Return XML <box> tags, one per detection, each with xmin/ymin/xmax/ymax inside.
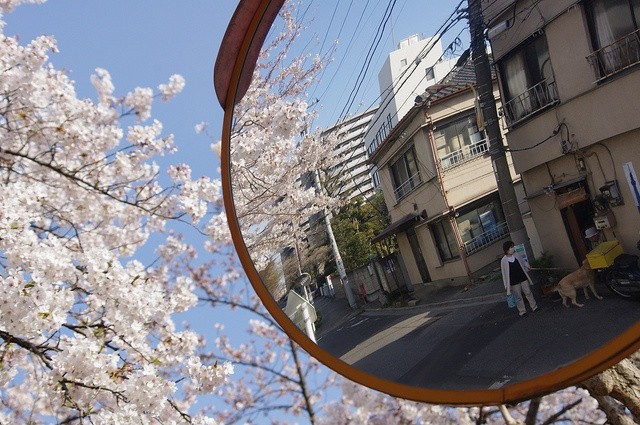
<box><xmin>542</xmin><ymin>256</ymin><xmax>603</xmax><ymax>307</ymax></box>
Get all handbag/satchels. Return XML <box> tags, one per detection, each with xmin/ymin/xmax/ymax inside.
<box><xmin>506</xmin><ymin>294</ymin><xmax>517</xmax><ymax>308</ymax></box>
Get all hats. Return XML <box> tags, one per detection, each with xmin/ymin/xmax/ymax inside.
<box><xmin>584</xmin><ymin>227</ymin><xmax>599</xmax><ymax>238</ymax></box>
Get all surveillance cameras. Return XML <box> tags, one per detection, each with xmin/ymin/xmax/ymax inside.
<box><xmin>414</xmin><ymin>91</ymin><xmax>431</xmax><ymax>107</ymax></box>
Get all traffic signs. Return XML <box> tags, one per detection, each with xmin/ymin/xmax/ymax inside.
<box><xmin>285</xmin><ymin>289</ymin><xmax>317</xmax><ymax>329</ymax></box>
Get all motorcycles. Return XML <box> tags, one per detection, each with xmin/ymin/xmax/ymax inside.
<box><xmin>601</xmin><ymin>253</ymin><xmax>640</xmax><ymax>299</ymax></box>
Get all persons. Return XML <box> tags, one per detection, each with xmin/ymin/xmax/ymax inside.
<box><xmin>584</xmin><ymin>227</ymin><xmax>606</xmax><ymax>249</ymax></box>
<box><xmin>499</xmin><ymin>239</ymin><xmax>541</xmax><ymax>318</ymax></box>
